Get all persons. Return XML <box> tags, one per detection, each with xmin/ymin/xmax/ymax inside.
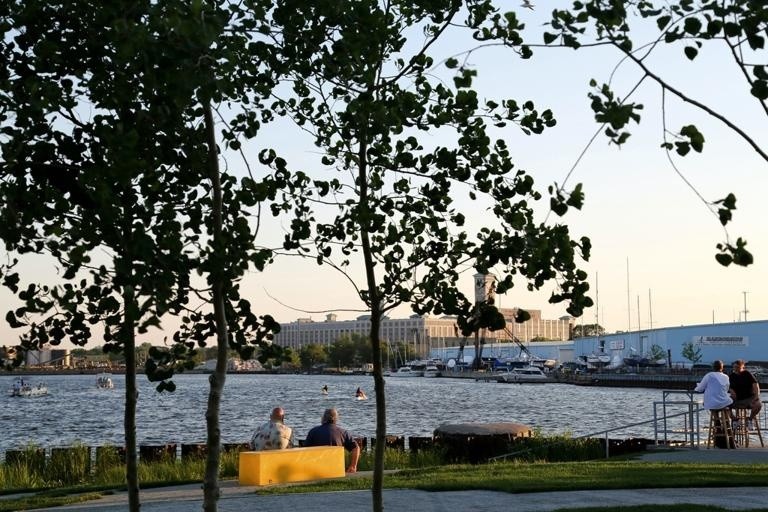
<box><xmin>695</xmin><ymin>359</ymin><xmax>738</xmax><ymax>427</ymax></box>
<box><xmin>15</xmin><ymin>381</ymin><xmax>21</xmax><ymax>389</ymax></box>
<box><xmin>356</xmin><ymin>386</ymin><xmax>365</xmax><ymax>396</ymax></box>
<box><xmin>248</xmin><ymin>406</ymin><xmax>292</xmax><ymax>450</ymax></box>
<box><xmin>323</xmin><ymin>384</ymin><xmax>328</xmax><ymax>391</ymax></box>
<box><xmin>305</xmin><ymin>408</ymin><xmax>360</xmax><ymax>473</ymax></box>
<box><xmin>724</xmin><ymin>359</ymin><xmax>761</xmax><ymax>431</ymax></box>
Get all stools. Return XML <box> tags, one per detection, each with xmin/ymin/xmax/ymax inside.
<box><xmin>706</xmin><ymin>407</ymin><xmax>765</xmax><ymax>450</ymax></box>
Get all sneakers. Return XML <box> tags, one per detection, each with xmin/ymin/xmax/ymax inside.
<box><xmin>732</xmin><ymin>417</ymin><xmax>755</xmax><ymax>431</ymax></box>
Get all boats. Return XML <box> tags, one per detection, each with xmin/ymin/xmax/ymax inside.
<box><xmin>381</xmin><ymin>366</ymin><xmax>440</xmax><ymax>378</ymax></box>
<box><xmin>8</xmin><ymin>379</ymin><xmax>47</xmax><ymax>397</ymax></box>
<box><xmin>97</xmin><ymin>377</ymin><xmax>113</xmax><ymax>389</ymax></box>
<box><xmin>492</xmin><ymin>367</ymin><xmax>553</xmax><ymax>383</ymax></box>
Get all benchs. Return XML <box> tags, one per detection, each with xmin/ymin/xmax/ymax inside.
<box><xmin>238</xmin><ymin>444</ymin><xmax>345</xmax><ymax>485</ymax></box>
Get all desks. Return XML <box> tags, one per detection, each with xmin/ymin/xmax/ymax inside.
<box><xmin>663</xmin><ymin>391</ymin><xmax>708</xmax><ymax>448</ymax></box>
<box><xmin>654</xmin><ymin>401</ymin><xmax>703</xmax><ymax>447</ymax></box>
